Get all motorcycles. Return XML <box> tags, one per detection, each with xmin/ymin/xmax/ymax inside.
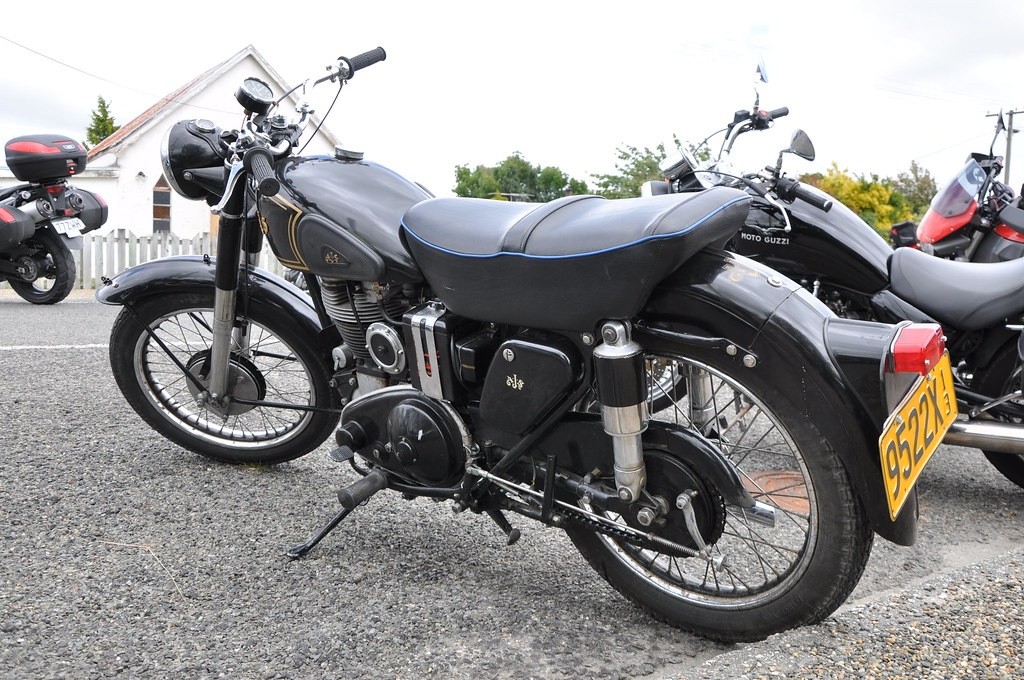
<box><xmin>0</xmin><ymin>135</ymin><xmax>108</xmax><ymax>305</ymax></box>
<box><xmin>893</xmin><ymin>105</ymin><xmax>1024</xmax><ymax>264</ymax></box>
<box><xmin>644</xmin><ymin>49</ymin><xmax>1024</xmax><ymax>497</ymax></box>
<box><xmin>97</xmin><ymin>45</ymin><xmax>960</xmax><ymax>647</ymax></box>
